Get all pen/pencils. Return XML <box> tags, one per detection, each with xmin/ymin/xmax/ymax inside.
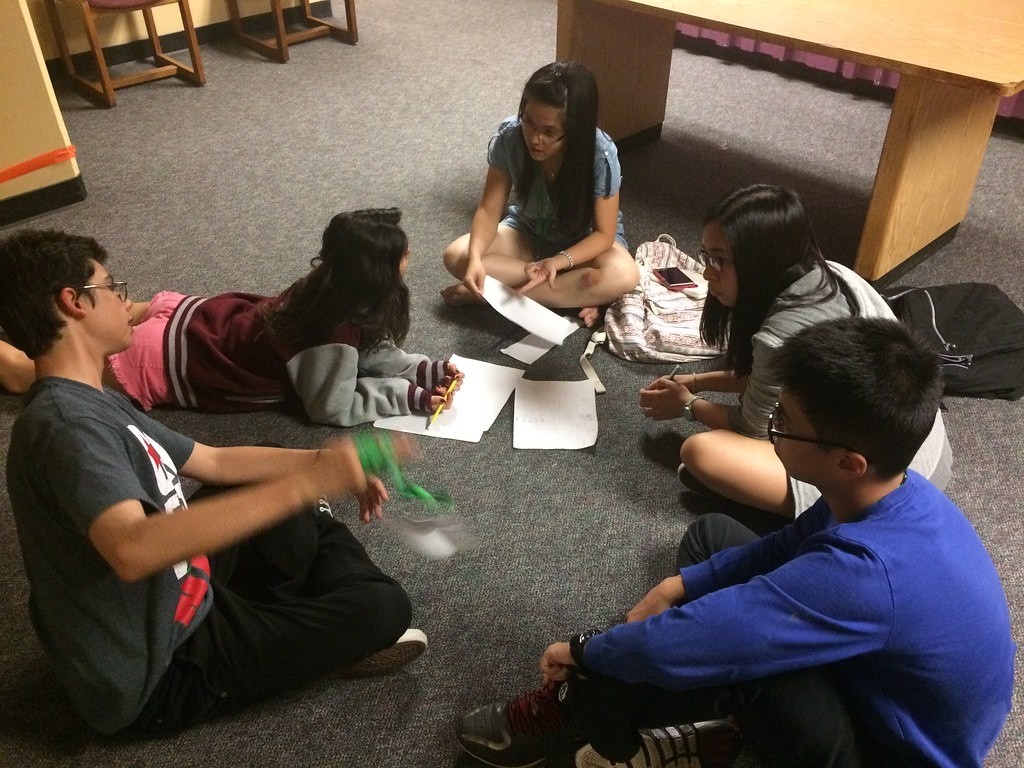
<box><xmin>669</xmin><ymin>364</ymin><xmax>680</xmax><ymax>381</ymax></box>
<box><xmin>426</xmin><ymin>379</ymin><xmax>457</xmax><ymax>428</ymax></box>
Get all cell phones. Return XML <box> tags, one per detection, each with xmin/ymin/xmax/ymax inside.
<box><xmin>658</xmin><ymin>267</ymin><xmax>694</xmax><ymax>286</ymax></box>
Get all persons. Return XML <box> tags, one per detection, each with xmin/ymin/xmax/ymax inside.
<box><xmin>0</xmin><ymin>206</ymin><xmax>464</xmax><ymax>426</ymax></box>
<box><xmin>639</xmin><ymin>184</ymin><xmax>943</xmax><ymax>520</ymax></box>
<box><xmin>451</xmin><ymin>317</ymin><xmax>1018</xmax><ymax>768</ymax></box>
<box><xmin>442</xmin><ymin>62</ymin><xmax>639</xmax><ymax>327</ymax></box>
<box><xmin>0</xmin><ymin>230</ymin><xmax>428</xmax><ymax>744</ymax></box>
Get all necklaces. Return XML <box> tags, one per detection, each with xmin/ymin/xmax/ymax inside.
<box><xmin>552</xmin><ymin>173</ymin><xmax>554</xmax><ymax>176</ymax></box>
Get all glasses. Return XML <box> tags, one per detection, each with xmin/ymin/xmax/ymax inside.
<box><xmin>518</xmin><ymin>112</ymin><xmax>568</xmax><ymax>142</ymax></box>
<box><xmin>697</xmin><ymin>249</ymin><xmax>737</xmax><ymax>272</ymax></box>
<box><xmin>768</xmin><ymin>402</ymin><xmax>872</xmax><ymax>466</ymax></box>
<box><xmin>57</xmin><ymin>280</ymin><xmax>129</xmax><ymax>302</ymax></box>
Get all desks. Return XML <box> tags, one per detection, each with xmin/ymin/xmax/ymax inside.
<box><xmin>554</xmin><ymin>0</ymin><xmax>1023</xmax><ymax>282</ymax></box>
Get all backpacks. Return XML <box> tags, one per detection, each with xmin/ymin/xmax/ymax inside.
<box><xmin>876</xmin><ymin>282</ymin><xmax>1024</xmax><ymax>400</ymax></box>
<box><xmin>579</xmin><ymin>234</ymin><xmax>737</xmax><ymax>393</ymax></box>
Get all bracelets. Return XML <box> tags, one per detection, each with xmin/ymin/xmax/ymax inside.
<box><xmin>558</xmin><ymin>251</ymin><xmax>573</xmax><ymax>268</ymax></box>
<box><xmin>694</xmin><ymin>373</ymin><xmax>696</xmax><ymax>393</ymax></box>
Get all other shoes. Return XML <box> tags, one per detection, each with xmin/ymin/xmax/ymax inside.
<box><xmin>312</xmin><ymin>492</ymin><xmax>332</xmax><ymax>518</ymax></box>
<box><xmin>678</xmin><ymin>463</ymin><xmax>723</xmax><ymax>498</ymax></box>
<box><xmin>319</xmin><ymin>628</ymin><xmax>428</xmax><ymax>678</ymax></box>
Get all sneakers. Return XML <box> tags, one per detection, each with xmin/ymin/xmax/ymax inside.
<box><xmin>574</xmin><ymin>720</ymin><xmax>742</xmax><ymax>768</ymax></box>
<box><xmin>454</xmin><ymin>685</ymin><xmax>587</xmax><ymax>768</ymax></box>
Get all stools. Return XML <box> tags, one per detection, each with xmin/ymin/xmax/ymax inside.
<box><xmin>44</xmin><ymin>0</ymin><xmax>203</xmax><ymax>105</ymax></box>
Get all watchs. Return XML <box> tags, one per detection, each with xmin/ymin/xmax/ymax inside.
<box><xmin>570</xmin><ymin>630</ymin><xmax>600</xmax><ymax>664</ymax></box>
<box><xmin>684</xmin><ymin>396</ymin><xmax>703</xmax><ymax>422</ymax></box>
